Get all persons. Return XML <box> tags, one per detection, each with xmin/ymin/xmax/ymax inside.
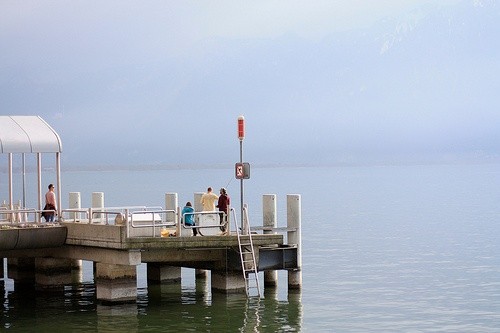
<box><xmin>214</xmin><ymin>188</ymin><xmax>230</xmax><ymax>235</ymax></box>
<box><xmin>200</xmin><ymin>186</ymin><xmax>219</xmax><ymax>222</ymax></box>
<box><xmin>180</xmin><ymin>201</ymin><xmax>198</xmax><ymax>237</ymax></box>
<box><xmin>40</xmin><ymin>184</ymin><xmax>58</xmax><ymax>222</ymax></box>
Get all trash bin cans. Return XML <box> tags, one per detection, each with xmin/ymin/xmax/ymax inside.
<box><xmin>114</xmin><ymin>212</ymin><xmax>162</xmax><ymax>238</ymax></box>
